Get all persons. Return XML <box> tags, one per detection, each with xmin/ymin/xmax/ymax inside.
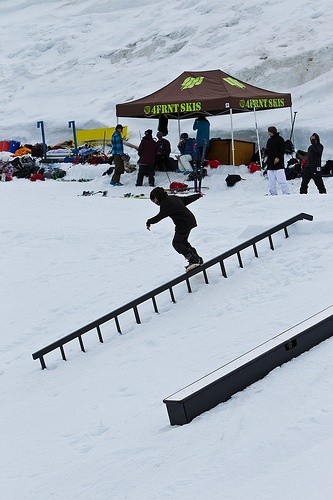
<box><xmin>110</xmin><ymin>124</ymin><xmax>125</xmax><ymax>186</ymax></box>
<box><xmin>146</xmin><ymin>187</ymin><xmax>206</xmax><ymax>269</ymax></box>
<box><xmin>135</xmin><ymin>130</ymin><xmax>158</xmax><ymax>186</ymax></box>
<box><xmin>154</xmin><ymin>132</ymin><xmax>171</xmax><ymax>172</ymax></box>
<box><xmin>264</xmin><ymin>127</ymin><xmax>291</xmax><ymax>196</ymax></box>
<box><xmin>193</xmin><ymin>115</ymin><xmax>210</xmax><ymax>166</ymax></box>
<box><xmin>300</xmin><ymin>132</ymin><xmax>326</xmax><ymax>194</ymax></box>
<box><xmin>177</xmin><ymin>133</ymin><xmax>196</xmax><ymax>173</ymax></box>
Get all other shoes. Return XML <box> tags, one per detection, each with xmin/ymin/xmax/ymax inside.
<box><xmin>185</xmin><ymin>249</ymin><xmax>203</xmax><ymax>272</ymax></box>
<box><xmin>264</xmin><ymin>193</ymin><xmax>291</xmax><ymax>196</ymax></box>
<box><xmin>110</xmin><ymin>182</ymin><xmax>124</xmax><ymax>187</ymax></box>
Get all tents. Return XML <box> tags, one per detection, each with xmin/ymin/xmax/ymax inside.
<box><xmin>116</xmin><ymin>69</ymin><xmax>296</xmax><ymax>175</ymax></box>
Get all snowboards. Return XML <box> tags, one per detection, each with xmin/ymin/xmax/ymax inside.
<box><xmin>187</xmin><ymin>264</ymin><xmax>200</xmax><ymax>272</ymax></box>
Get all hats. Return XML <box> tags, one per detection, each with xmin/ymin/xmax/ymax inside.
<box><xmin>116</xmin><ymin>124</ymin><xmax>125</xmax><ymax>129</ymax></box>
